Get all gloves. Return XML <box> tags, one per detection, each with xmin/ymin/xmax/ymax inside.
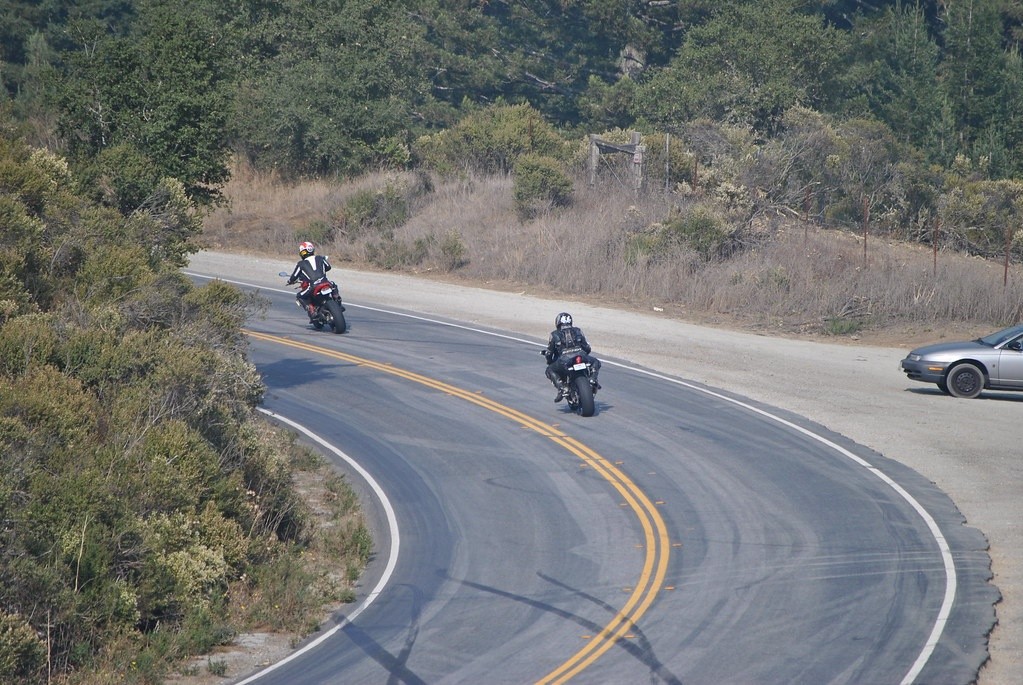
<box><xmin>287</xmin><ymin>279</ymin><xmax>292</xmax><ymax>284</ymax></box>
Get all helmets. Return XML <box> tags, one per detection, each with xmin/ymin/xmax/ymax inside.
<box><xmin>299</xmin><ymin>242</ymin><xmax>315</xmax><ymax>256</ymax></box>
<box><xmin>555</xmin><ymin>312</ymin><xmax>572</xmax><ymax>329</ymax></box>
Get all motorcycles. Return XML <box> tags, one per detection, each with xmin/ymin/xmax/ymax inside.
<box><xmin>279</xmin><ymin>256</ymin><xmax>346</xmax><ymax>334</ymax></box>
<box><xmin>540</xmin><ymin>350</ymin><xmax>601</xmax><ymax>418</ymax></box>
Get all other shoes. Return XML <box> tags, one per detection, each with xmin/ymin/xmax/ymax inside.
<box><xmin>553</xmin><ymin>386</ymin><xmax>571</xmax><ymax>403</ymax></box>
<box><xmin>592</xmin><ymin>379</ymin><xmax>602</xmax><ymax>390</ymax></box>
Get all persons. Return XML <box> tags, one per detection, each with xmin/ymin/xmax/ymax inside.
<box><xmin>545</xmin><ymin>313</ymin><xmax>601</xmax><ymax>402</ymax></box>
<box><xmin>289</xmin><ymin>242</ymin><xmax>345</xmax><ymax>324</ymax></box>
